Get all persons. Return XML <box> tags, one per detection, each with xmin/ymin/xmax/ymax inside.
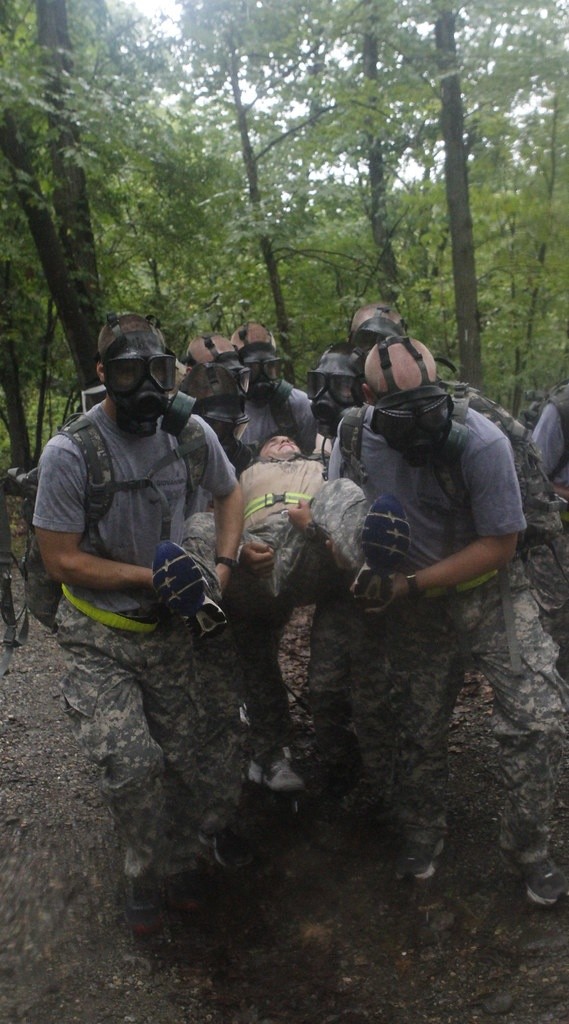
<box><xmin>329</xmin><ymin>335</ymin><xmax>568</xmax><ymax>905</ymax></box>
<box><xmin>151</xmin><ymin>436</ymin><xmax>412</xmax><ymax>641</ymax></box>
<box><xmin>175</xmin><ymin>304</ymin><xmax>568</xmax><ymax>869</ymax></box>
<box><xmin>31</xmin><ymin>312</ymin><xmax>245</xmax><ymax>935</ymax></box>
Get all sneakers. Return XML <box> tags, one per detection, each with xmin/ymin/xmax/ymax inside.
<box><xmin>396</xmin><ymin>838</ymin><xmax>445</xmax><ymax>877</ymax></box>
<box><xmin>151</xmin><ymin>542</ymin><xmax>228</xmax><ymax>639</ymax></box>
<box><xmin>247</xmin><ymin>762</ymin><xmax>305</xmax><ymax>789</ymax></box>
<box><xmin>126</xmin><ymin>909</ymin><xmax>168</xmax><ymax>933</ymax></box>
<box><xmin>355</xmin><ymin>495</ymin><xmax>412</xmax><ymax>611</ymax></box>
<box><xmin>199</xmin><ymin>829</ymin><xmax>255</xmax><ymax>867</ymax></box>
<box><xmin>160</xmin><ymin>887</ymin><xmax>201</xmax><ymax>912</ymax></box>
<box><xmin>504</xmin><ymin>852</ymin><xmax>568</xmax><ymax>905</ymax></box>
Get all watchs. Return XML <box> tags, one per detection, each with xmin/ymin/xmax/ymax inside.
<box><xmin>406</xmin><ymin>571</ymin><xmax>420</xmax><ymax>600</ymax></box>
<box><xmin>305</xmin><ymin>523</ymin><xmax>318</xmax><ymax>540</ymax></box>
<box><xmin>216</xmin><ymin>557</ymin><xmax>238</xmax><ymax>573</ymax></box>
